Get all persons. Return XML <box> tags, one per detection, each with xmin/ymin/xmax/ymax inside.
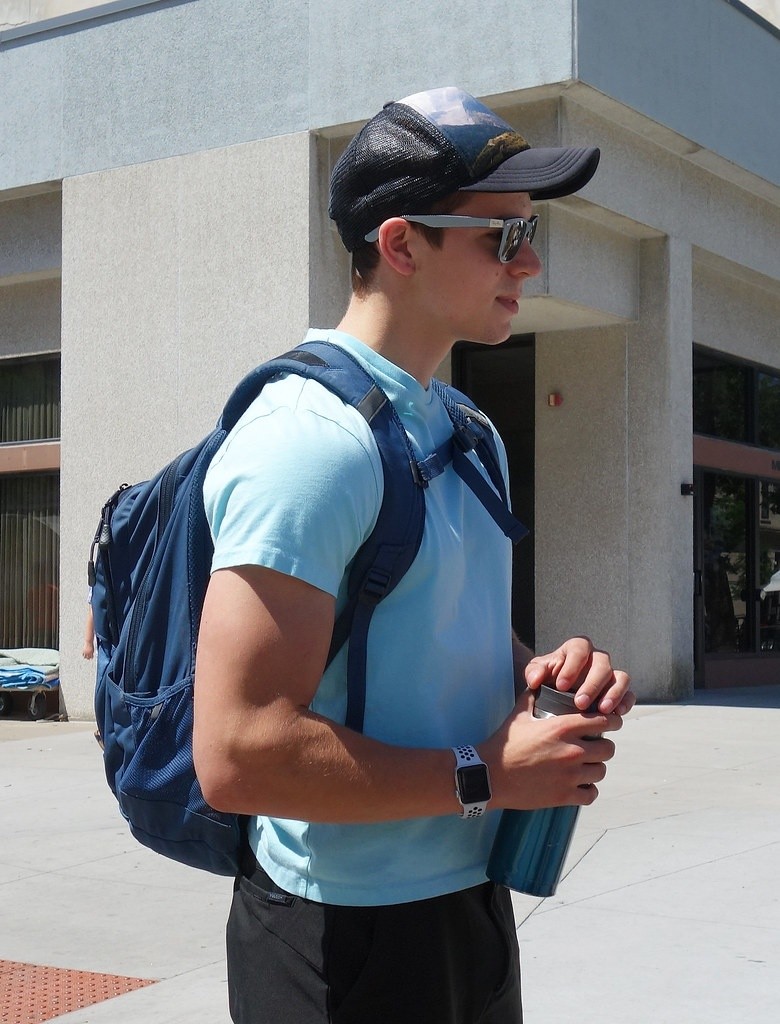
<box><xmin>183</xmin><ymin>86</ymin><xmax>636</xmax><ymax>1022</ymax></box>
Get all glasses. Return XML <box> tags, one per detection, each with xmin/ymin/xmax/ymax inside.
<box><xmin>363</xmin><ymin>214</ymin><xmax>541</xmax><ymax>263</ymax></box>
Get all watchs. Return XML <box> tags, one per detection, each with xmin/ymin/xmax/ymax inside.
<box><xmin>451</xmin><ymin>743</ymin><xmax>493</xmax><ymax>821</ymax></box>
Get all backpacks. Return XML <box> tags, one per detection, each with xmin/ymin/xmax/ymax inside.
<box><xmin>90</xmin><ymin>342</ymin><xmax>529</xmax><ymax>879</ymax></box>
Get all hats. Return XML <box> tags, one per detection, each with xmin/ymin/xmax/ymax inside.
<box><xmin>328</xmin><ymin>86</ymin><xmax>600</xmax><ymax>251</ymax></box>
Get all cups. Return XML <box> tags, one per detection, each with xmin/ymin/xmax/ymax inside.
<box><xmin>484</xmin><ymin>680</ymin><xmax>602</xmax><ymax>897</ymax></box>
